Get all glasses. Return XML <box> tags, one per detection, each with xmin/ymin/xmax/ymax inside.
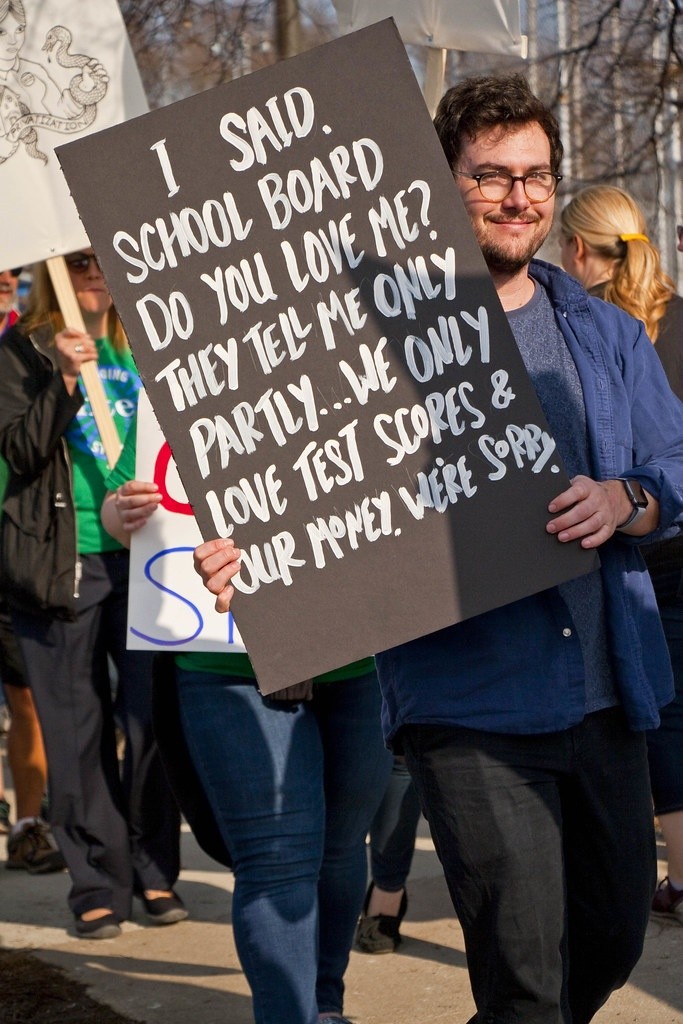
<box><xmin>67</xmin><ymin>252</ymin><xmax>102</xmax><ymax>274</ymax></box>
<box><xmin>11</xmin><ymin>267</ymin><xmax>22</xmax><ymax>277</ymax></box>
<box><xmin>450</xmin><ymin>169</ymin><xmax>564</xmax><ymax>203</ymax></box>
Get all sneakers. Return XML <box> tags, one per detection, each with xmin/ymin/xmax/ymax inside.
<box><xmin>7</xmin><ymin>822</ymin><xmax>66</xmax><ymax>874</ymax></box>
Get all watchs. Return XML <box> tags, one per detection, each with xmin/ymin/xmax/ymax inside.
<box><xmin>614</xmin><ymin>477</ymin><xmax>649</xmax><ymax>532</ymax></box>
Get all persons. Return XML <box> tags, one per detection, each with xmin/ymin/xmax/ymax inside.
<box><xmin>102</xmin><ymin>412</ymin><xmax>394</xmax><ymax>1024</ymax></box>
<box><xmin>193</xmin><ymin>74</ymin><xmax>683</xmax><ymax>1024</ymax></box>
<box><xmin>0</xmin><ymin>248</ymin><xmax>420</xmax><ymax>953</ymax></box>
<box><xmin>558</xmin><ymin>186</ymin><xmax>683</xmax><ymax>928</ymax></box>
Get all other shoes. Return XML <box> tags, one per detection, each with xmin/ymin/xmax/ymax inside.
<box><xmin>135</xmin><ymin>886</ymin><xmax>188</xmax><ymax>923</ymax></box>
<box><xmin>77</xmin><ymin>909</ymin><xmax>121</xmax><ymax>939</ymax></box>
<box><xmin>357</xmin><ymin>880</ymin><xmax>408</xmax><ymax>955</ymax></box>
<box><xmin>650</xmin><ymin>878</ymin><xmax>683</xmax><ymax>919</ymax></box>
